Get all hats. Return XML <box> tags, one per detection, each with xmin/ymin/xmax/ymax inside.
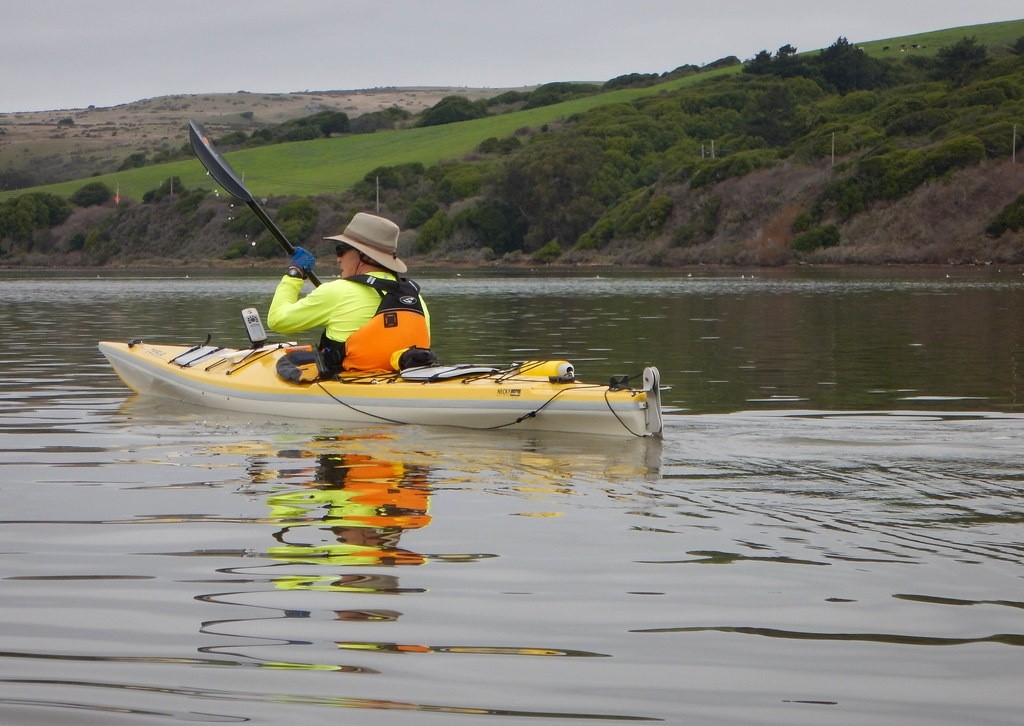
<box><xmin>323</xmin><ymin>212</ymin><xmax>407</xmax><ymax>273</ymax></box>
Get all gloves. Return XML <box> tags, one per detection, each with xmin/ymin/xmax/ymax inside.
<box><xmin>288</xmin><ymin>245</ymin><xmax>317</xmax><ymax>279</ymax></box>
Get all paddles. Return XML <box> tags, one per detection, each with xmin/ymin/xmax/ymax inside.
<box><xmin>183</xmin><ymin>115</ymin><xmax>324</xmax><ymax>288</ymax></box>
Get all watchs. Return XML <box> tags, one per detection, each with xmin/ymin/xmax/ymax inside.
<box><xmin>282</xmin><ymin>268</ymin><xmax>305</xmax><ymax>280</ymax></box>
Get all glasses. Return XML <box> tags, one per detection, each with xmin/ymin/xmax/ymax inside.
<box><xmin>336</xmin><ymin>244</ymin><xmax>354</xmax><ymax>257</ymax></box>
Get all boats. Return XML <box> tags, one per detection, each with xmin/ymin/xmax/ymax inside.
<box><xmin>99</xmin><ymin>307</ymin><xmax>671</xmax><ymax>437</ymax></box>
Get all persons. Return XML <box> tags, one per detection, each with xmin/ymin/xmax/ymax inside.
<box><xmin>267</xmin><ymin>212</ymin><xmax>431</xmax><ymax>370</ymax></box>
